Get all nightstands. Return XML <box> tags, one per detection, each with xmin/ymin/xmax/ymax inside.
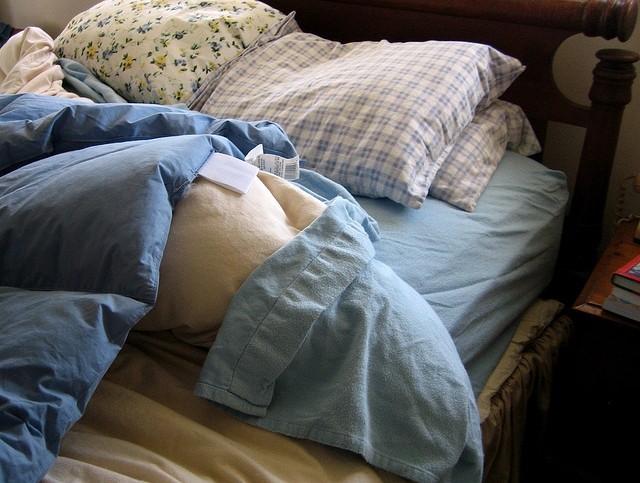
<box><xmin>573</xmin><ymin>220</ymin><xmax>638</xmax><ymax>330</ymax></box>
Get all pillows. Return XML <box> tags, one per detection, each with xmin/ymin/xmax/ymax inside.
<box><xmin>53</xmin><ymin>1</ymin><xmax>289</xmax><ymax>105</ymax></box>
<box><xmin>186</xmin><ymin>11</ymin><xmax>527</xmax><ymax>211</ymax></box>
<box><xmin>427</xmin><ymin>98</ymin><xmax>541</xmax><ymax>210</ymax></box>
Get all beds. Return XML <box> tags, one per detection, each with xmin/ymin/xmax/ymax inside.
<box><xmin>0</xmin><ymin>0</ymin><xmax>638</xmax><ymax>481</ymax></box>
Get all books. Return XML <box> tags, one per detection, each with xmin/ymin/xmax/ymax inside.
<box><xmin>601</xmin><ymin>295</ymin><xmax>640</xmax><ymax>322</ymax></box>
<box><xmin>610</xmin><ymin>253</ymin><xmax>640</xmax><ymax>296</ymax></box>
<box><xmin>610</xmin><ymin>285</ymin><xmax>640</xmax><ymax>307</ymax></box>
